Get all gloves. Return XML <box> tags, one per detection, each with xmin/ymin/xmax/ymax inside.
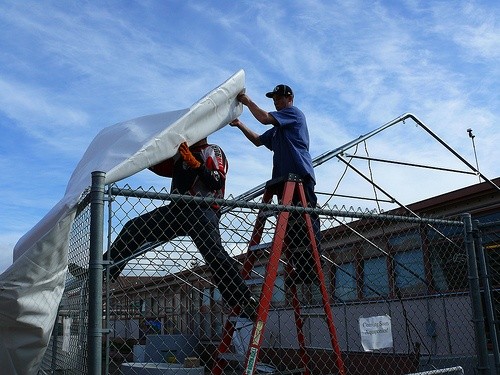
<box><xmin>179</xmin><ymin>142</ymin><xmax>200</xmax><ymax>170</ymax></box>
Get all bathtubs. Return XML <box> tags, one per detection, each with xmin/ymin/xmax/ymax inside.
<box><xmin>121</xmin><ymin>363</ymin><xmax>203</xmax><ymax>375</ymax></box>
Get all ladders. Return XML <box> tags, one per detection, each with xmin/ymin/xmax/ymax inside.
<box><xmin>206</xmin><ymin>173</ymin><xmax>348</xmax><ymax>375</ymax></box>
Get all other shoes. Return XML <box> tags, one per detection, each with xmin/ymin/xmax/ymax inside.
<box><xmin>67</xmin><ymin>263</ymin><xmax>90</xmax><ymax>282</ymax></box>
<box><xmin>285</xmin><ymin>271</ymin><xmax>320</xmax><ymax>286</ymax></box>
<box><xmin>228</xmin><ymin>306</ymin><xmax>257</xmax><ymax>322</ymax></box>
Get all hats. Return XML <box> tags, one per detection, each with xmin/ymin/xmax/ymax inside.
<box><xmin>266</xmin><ymin>84</ymin><xmax>293</xmax><ymax>99</ymax></box>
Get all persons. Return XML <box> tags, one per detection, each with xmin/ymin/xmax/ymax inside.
<box><xmin>229</xmin><ymin>85</ymin><xmax>326</xmax><ymax>286</ymax></box>
<box><xmin>68</xmin><ymin>136</ymin><xmax>259</xmax><ymax>318</ymax></box>
